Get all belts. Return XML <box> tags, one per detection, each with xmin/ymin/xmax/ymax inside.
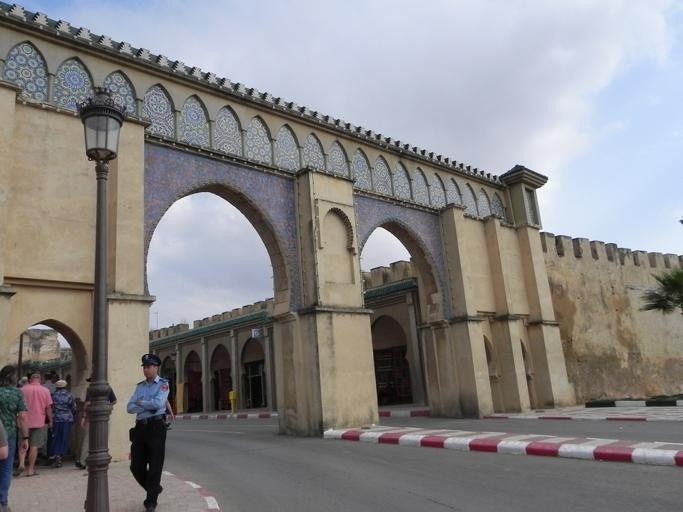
<box><xmin>134</xmin><ymin>414</ymin><xmax>166</xmax><ymax>425</ymax></box>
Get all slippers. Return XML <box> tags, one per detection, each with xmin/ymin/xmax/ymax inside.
<box><xmin>12</xmin><ymin>466</ymin><xmax>41</xmax><ymax>478</ymax></box>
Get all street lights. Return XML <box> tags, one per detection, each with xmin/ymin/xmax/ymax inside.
<box><xmin>75</xmin><ymin>86</ymin><xmax>129</xmax><ymax>512</ymax></box>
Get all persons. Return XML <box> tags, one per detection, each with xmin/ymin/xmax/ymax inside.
<box><xmin>0</xmin><ymin>363</ymin><xmax>117</xmax><ymax>512</ymax></box>
<box><xmin>125</xmin><ymin>352</ymin><xmax>172</xmax><ymax>512</ymax></box>
<box><xmin>163</xmin><ymin>399</ymin><xmax>176</xmax><ymax>424</ymax></box>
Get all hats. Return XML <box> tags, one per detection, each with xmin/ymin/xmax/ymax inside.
<box><xmin>140</xmin><ymin>354</ymin><xmax>163</xmax><ymax>367</ymax></box>
<box><xmin>55</xmin><ymin>380</ymin><xmax>67</xmax><ymax>388</ymax></box>
